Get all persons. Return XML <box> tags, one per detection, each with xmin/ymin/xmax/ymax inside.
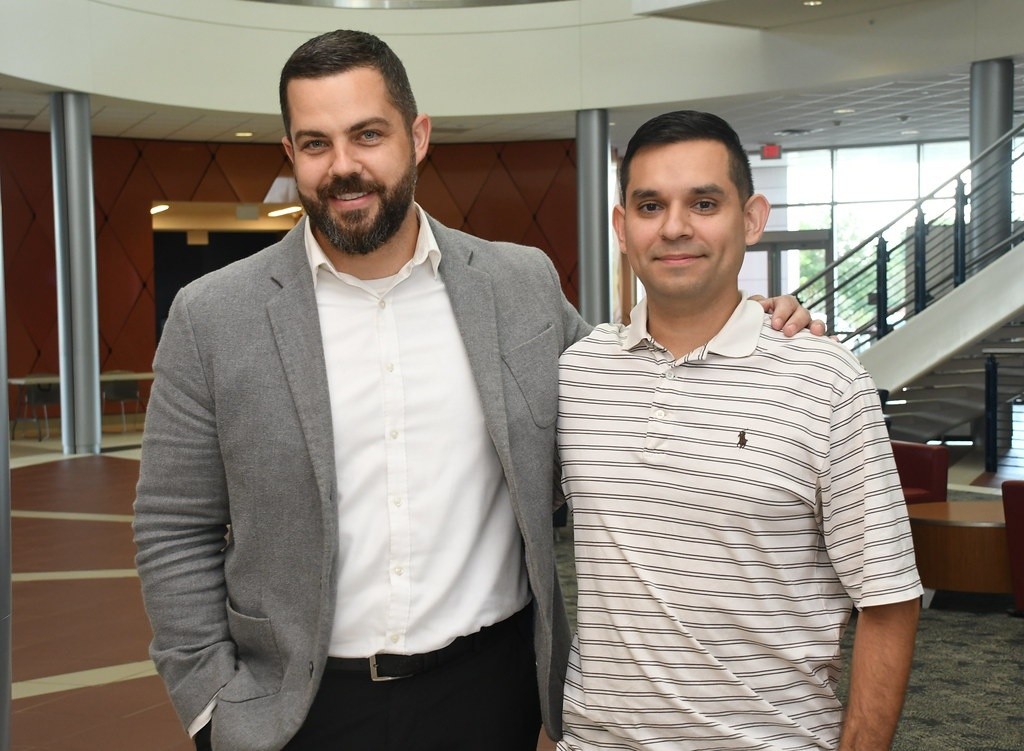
<box><xmin>132</xmin><ymin>29</ymin><xmax>838</xmax><ymax>751</ymax></box>
<box><xmin>558</xmin><ymin>111</ymin><xmax>925</xmax><ymax>751</ymax></box>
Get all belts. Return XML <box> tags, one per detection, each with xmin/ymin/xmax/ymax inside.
<box><xmin>325</xmin><ymin>603</ymin><xmax>534</xmax><ymax>682</ymax></box>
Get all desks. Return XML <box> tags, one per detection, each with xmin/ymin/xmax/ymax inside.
<box><xmin>904</xmin><ymin>499</ymin><xmax>1008</xmax><ymax>612</ymax></box>
<box><xmin>9</xmin><ymin>372</ymin><xmax>155</xmax><ymax>444</ymax></box>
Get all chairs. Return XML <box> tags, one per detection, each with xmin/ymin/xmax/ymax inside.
<box><xmin>890</xmin><ymin>441</ymin><xmax>1024</xmax><ymax>615</ymax></box>
<box><xmin>24</xmin><ymin>381</ymin><xmax>61</xmax><ymax>440</ymax></box>
<box><xmin>103</xmin><ymin>371</ymin><xmax>146</xmax><ymax>433</ymax></box>
